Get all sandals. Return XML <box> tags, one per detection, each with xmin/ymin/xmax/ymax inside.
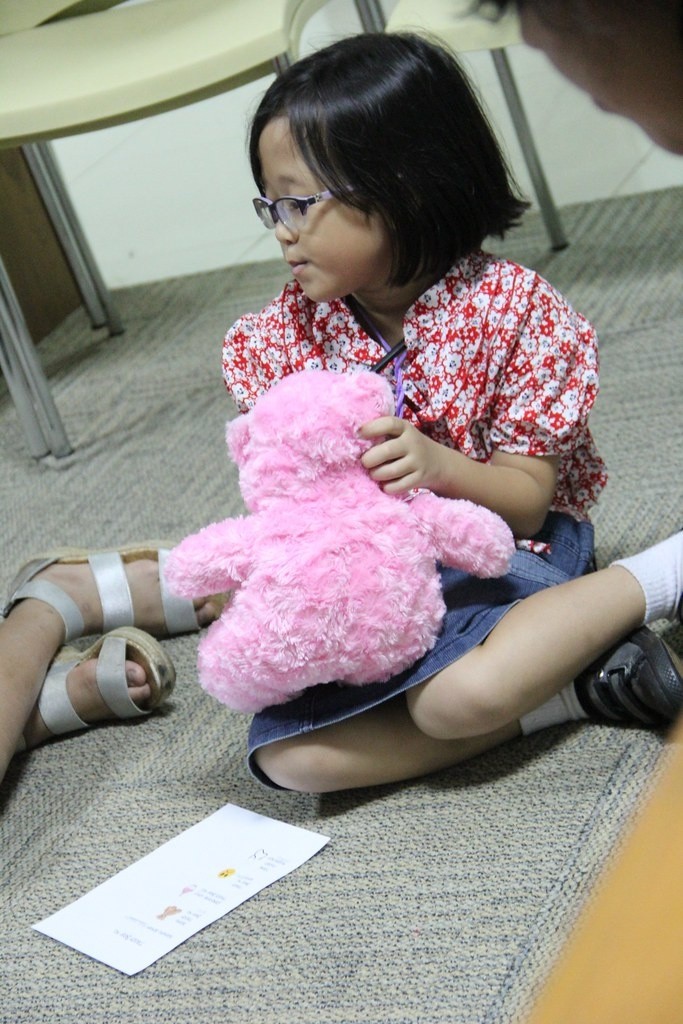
<box><xmin>3</xmin><ymin>539</ymin><xmax>226</xmax><ymax>646</ymax></box>
<box><xmin>14</xmin><ymin>625</ymin><xmax>177</xmax><ymax>752</ymax></box>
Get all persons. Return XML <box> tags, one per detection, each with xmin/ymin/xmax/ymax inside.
<box><xmin>221</xmin><ymin>32</ymin><xmax>683</xmax><ymax>792</ymax></box>
<box><xmin>0</xmin><ymin>540</ymin><xmax>232</xmax><ymax>783</ymax></box>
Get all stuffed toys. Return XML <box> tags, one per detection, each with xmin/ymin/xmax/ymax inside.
<box><xmin>164</xmin><ymin>369</ymin><xmax>515</xmax><ymax>713</ymax></box>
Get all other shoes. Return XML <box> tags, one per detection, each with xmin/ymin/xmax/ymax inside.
<box><xmin>574</xmin><ymin>626</ymin><xmax>683</xmax><ymax>732</ymax></box>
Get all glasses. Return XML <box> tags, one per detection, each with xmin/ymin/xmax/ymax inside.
<box><xmin>250</xmin><ymin>172</ymin><xmax>405</xmax><ymax>235</ymax></box>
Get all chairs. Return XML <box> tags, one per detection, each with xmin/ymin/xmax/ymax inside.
<box><xmin>0</xmin><ymin>0</ymin><xmax>572</xmax><ymax>459</ymax></box>
